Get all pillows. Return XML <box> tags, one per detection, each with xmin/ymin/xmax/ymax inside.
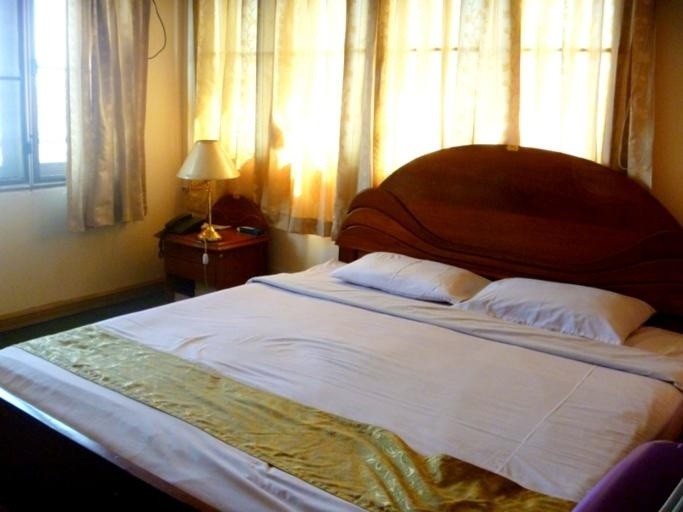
<box><xmin>328</xmin><ymin>247</ymin><xmax>488</xmax><ymax>306</ymax></box>
<box><xmin>455</xmin><ymin>274</ymin><xmax>656</xmax><ymax>347</ymax></box>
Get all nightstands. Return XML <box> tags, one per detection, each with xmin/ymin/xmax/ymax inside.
<box><xmin>154</xmin><ymin>225</ymin><xmax>278</xmax><ymax>297</ymax></box>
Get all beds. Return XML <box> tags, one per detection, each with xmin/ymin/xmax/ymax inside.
<box><xmin>0</xmin><ymin>142</ymin><xmax>681</xmax><ymax>512</ymax></box>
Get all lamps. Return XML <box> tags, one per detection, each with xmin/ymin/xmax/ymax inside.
<box><xmin>175</xmin><ymin>139</ymin><xmax>241</xmax><ymax>242</ymax></box>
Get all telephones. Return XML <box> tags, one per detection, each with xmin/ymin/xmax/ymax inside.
<box><xmin>166</xmin><ymin>213</ymin><xmax>204</xmax><ymax>234</ymax></box>
